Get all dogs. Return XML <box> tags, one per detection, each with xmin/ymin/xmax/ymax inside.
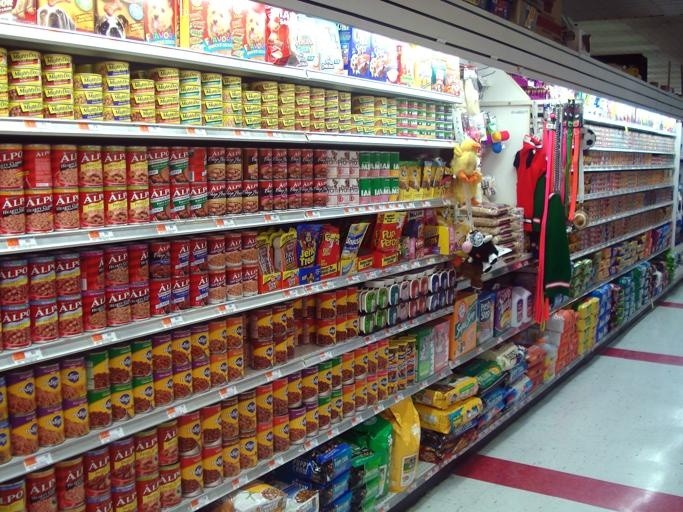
<box><xmin>245</xmin><ymin>8</ymin><xmax>266</xmax><ymax>44</ymax></box>
<box><xmin>95</xmin><ymin>14</ymin><xmax>128</xmax><ymax>40</ymax></box>
<box><xmin>207</xmin><ymin>0</ymin><xmax>231</xmax><ymax>36</ymax></box>
<box><xmin>36</xmin><ymin>6</ymin><xmax>76</xmax><ymax>32</ymax></box>
<box><xmin>351</xmin><ymin>53</ymin><xmax>359</xmax><ymax>65</ymax></box>
<box><xmin>146</xmin><ymin>0</ymin><xmax>172</xmax><ymax>33</ymax></box>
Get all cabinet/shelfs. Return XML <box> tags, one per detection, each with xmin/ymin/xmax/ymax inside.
<box><xmin>0</xmin><ymin>1</ymin><xmax>683</xmax><ymax>512</ymax></box>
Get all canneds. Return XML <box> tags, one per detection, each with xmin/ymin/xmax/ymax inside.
<box><xmin>0</xmin><ymin>46</ymin><xmax>454</xmax><ymax>139</ymax></box>
<box><xmin>1</xmin><ymin>337</ymin><xmax>416</xmax><ymax>512</ymax></box>
<box><xmin>0</xmin><ymin>144</ymin><xmax>400</xmax><ymax>236</ymax></box>
<box><xmin>0</xmin><ymin>230</ymin><xmax>259</xmax><ymax>353</ymax></box>
<box><xmin>1</xmin><ymin>290</ymin><xmax>358</xmax><ymax>464</ymax></box>
